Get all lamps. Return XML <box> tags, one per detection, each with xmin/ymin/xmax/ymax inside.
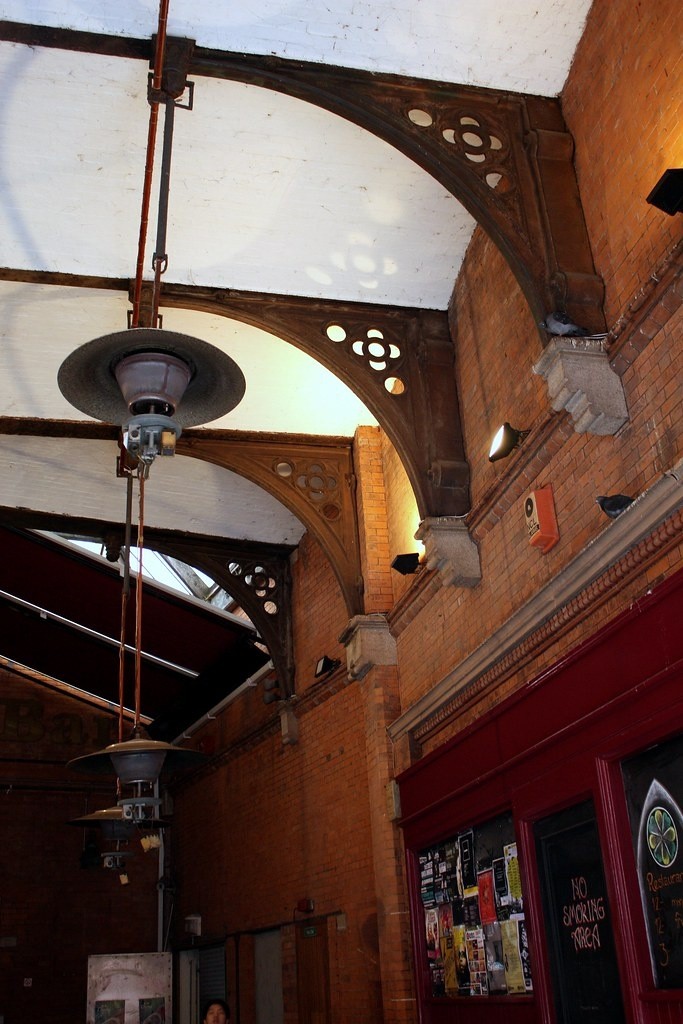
<box><xmin>645</xmin><ymin>168</ymin><xmax>683</xmax><ymax>217</ymax></box>
<box><xmin>391</xmin><ymin>553</ymin><xmax>427</xmax><ymax>575</ymax></box>
<box><xmin>488</xmin><ymin>422</ymin><xmax>531</xmax><ymax>463</ymax></box>
<box><xmin>314</xmin><ymin>655</ymin><xmax>341</xmax><ymax>678</ymax></box>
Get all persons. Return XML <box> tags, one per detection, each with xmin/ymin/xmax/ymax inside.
<box><xmin>202</xmin><ymin>999</ymin><xmax>231</xmax><ymax>1024</ymax></box>
